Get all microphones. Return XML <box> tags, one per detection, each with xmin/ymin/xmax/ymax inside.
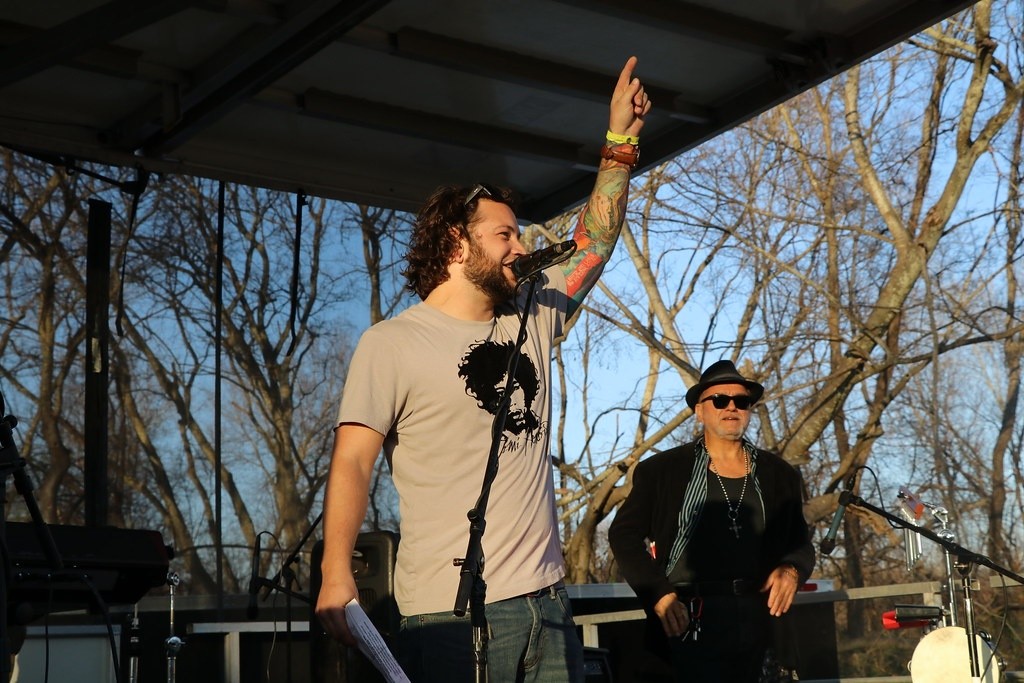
<box><xmin>512</xmin><ymin>240</ymin><xmax>575</xmax><ymax>277</ymax></box>
<box><xmin>820</xmin><ymin>474</ymin><xmax>857</xmax><ymax>555</ymax></box>
<box><xmin>247</xmin><ymin>535</ymin><xmax>261</xmax><ymax>618</ymax></box>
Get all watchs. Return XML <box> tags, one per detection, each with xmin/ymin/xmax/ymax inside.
<box><xmin>600</xmin><ymin>144</ymin><xmax>641</xmax><ymax>169</ymax></box>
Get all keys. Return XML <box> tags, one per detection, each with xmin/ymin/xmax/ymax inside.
<box><xmin>681</xmin><ymin>618</ymin><xmax>701</xmax><ymax>641</ymax></box>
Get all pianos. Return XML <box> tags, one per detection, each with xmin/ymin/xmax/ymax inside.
<box><xmin>0</xmin><ymin>521</ymin><xmax>170</xmax><ymax>616</ymax></box>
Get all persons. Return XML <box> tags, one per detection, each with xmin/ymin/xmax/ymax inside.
<box><xmin>315</xmin><ymin>56</ymin><xmax>651</xmax><ymax>683</ymax></box>
<box><xmin>607</xmin><ymin>361</ymin><xmax>816</xmax><ymax>682</ymax></box>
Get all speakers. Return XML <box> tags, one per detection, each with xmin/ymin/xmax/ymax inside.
<box><xmin>308</xmin><ymin>529</ymin><xmax>402</xmax><ymax>683</ymax></box>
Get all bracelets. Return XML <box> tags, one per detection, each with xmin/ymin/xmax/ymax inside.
<box><xmin>605</xmin><ymin>130</ymin><xmax>640</xmax><ymax>146</ymax></box>
<box><xmin>783</xmin><ymin>563</ymin><xmax>803</xmax><ymax>582</ymax></box>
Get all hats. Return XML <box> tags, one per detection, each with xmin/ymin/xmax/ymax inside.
<box><xmin>686</xmin><ymin>360</ymin><xmax>764</xmax><ymax>412</ymax></box>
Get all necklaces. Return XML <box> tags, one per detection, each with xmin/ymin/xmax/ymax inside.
<box><xmin>705</xmin><ymin>444</ymin><xmax>748</xmax><ymax>539</ymax></box>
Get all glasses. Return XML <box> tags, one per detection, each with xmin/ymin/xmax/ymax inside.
<box><xmin>465</xmin><ymin>184</ymin><xmax>492</xmax><ymax>204</ymax></box>
<box><xmin>701</xmin><ymin>393</ymin><xmax>751</xmax><ymax>410</ymax></box>
